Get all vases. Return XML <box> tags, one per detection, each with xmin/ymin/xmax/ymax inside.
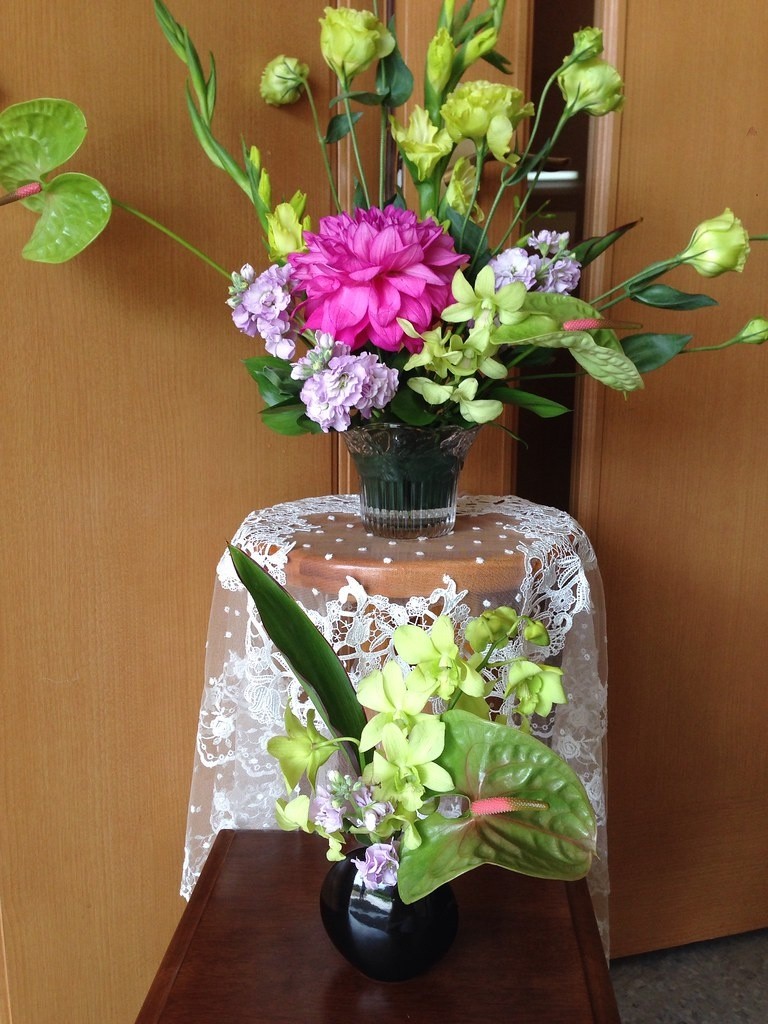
<box><xmin>332</xmin><ymin>420</ymin><xmax>488</xmax><ymax>539</ymax></box>
<box><xmin>319</xmin><ymin>846</ymin><xmax>465</xmax><ymax>982</ymax></box>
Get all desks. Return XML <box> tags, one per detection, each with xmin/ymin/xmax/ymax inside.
<box><xmin>134</xmin><ymin>828</ymin><xmax>616</xmax><ymax>1024</ymax></box>
<box><xmin>179</xmin><ymin>495</ymin><xmax>610</xmax><ymax>975</ymax></box>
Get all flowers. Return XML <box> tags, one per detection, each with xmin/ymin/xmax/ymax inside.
<box><xmin>0</xmin><ymin>0</ymin><xmax>768</xmax><ymax>507</ymax></box>
<box><xmin>223</xmin><ymin>539</ymin><xmax>599</xmax><ymax>903</ymax></box>
